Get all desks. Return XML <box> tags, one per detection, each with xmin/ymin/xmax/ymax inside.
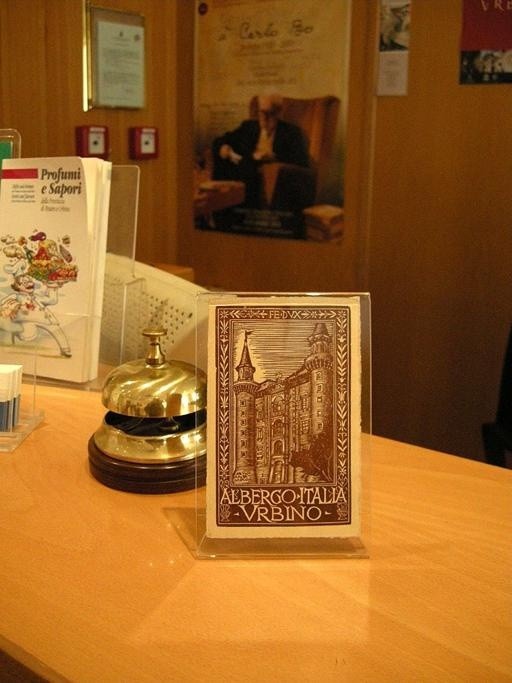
<box><xmin>192</xmin><ymin>177</ymin><xmax>245</xmax><ymax>215</ymax></box>
<box><xmin>1</xmin><ymin>357</ymin><xmax>511</xmax><ymax>682</ymax></box>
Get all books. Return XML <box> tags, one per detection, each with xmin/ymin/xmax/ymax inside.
<box><xmin>0</xmin><ymin>156</ymin><xmax>112</xmax><ymax>384</ymax></box>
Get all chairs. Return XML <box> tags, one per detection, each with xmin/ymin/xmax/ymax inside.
<box><xmin>102</xmin><ymin>252</ymin><xmax>208</xmax><ymax>373</ymax></box>
<box><xmin>249</xmin><ymin>93</ymin><xmax>342</xmax><ymax>200</ymax></box>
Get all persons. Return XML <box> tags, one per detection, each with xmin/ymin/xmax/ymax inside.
<box><xmin>213</xmin><ymin>84</ymin><xmax>310</xmax><ymax>208</ymax></box>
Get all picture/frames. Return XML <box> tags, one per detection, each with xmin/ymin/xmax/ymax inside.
<box><xmin>193</xmin><ymin>291</ymin><xmax>372</xmax><ymax>558</ymax></box>
